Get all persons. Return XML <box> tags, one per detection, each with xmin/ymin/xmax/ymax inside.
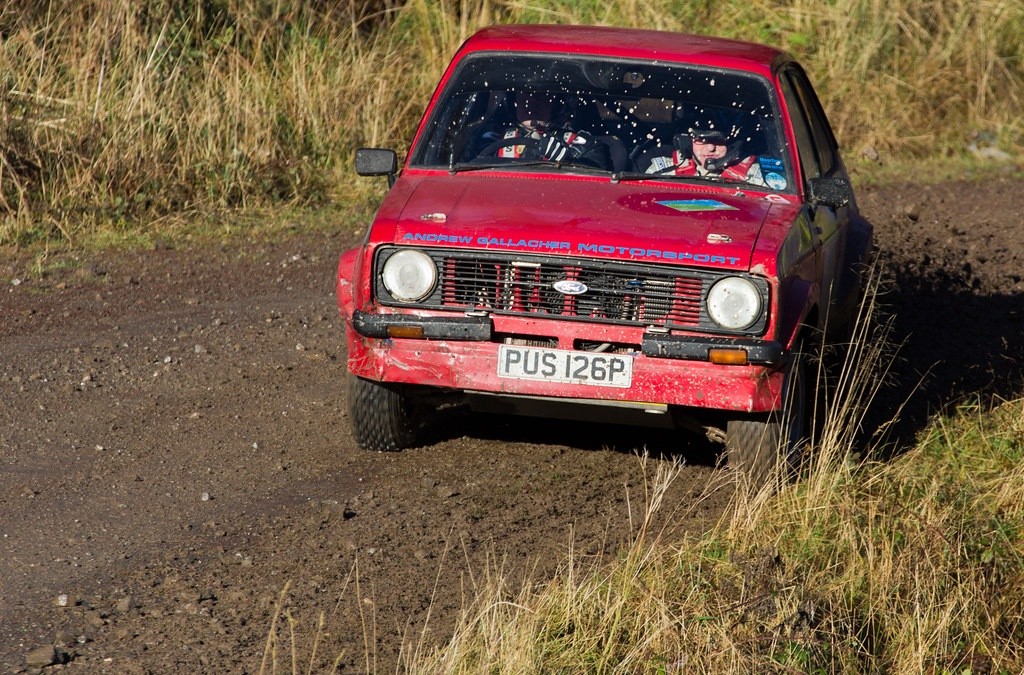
<box><xmin>647</xmin><ymin>106</ymin><xmax>770</xmax><ymax>189</ymax></box>
<box><xmin>497</xmin><ymin>70</ymin><xmax>614</xmax><ymax>173</ymax></box>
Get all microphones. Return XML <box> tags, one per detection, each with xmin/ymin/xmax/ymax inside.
<box><xmin>536</xmin><ymin>113</ymin><xmax>571</xmax><ymax>133</ymax></box>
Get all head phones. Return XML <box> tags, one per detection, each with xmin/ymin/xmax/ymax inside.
<box><xmin>673</xmin><ymin>119</ymin><xmax>752</xmax><ymax>161</ymax></box>
<box><xmin>705</xmin><ymin>137</ymin><xmax>751</xmax><ymax>171</ymax></box>
<box><xmin>504</xmin><ymin>88</ymin><xmax>574</xmax><ymax>125</ymax></box>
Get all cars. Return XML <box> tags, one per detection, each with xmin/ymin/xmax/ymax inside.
<box><xmin>334</xmin><ymin>26</ymin><xmax>871</xmax><ymax>495</ymax></box>
